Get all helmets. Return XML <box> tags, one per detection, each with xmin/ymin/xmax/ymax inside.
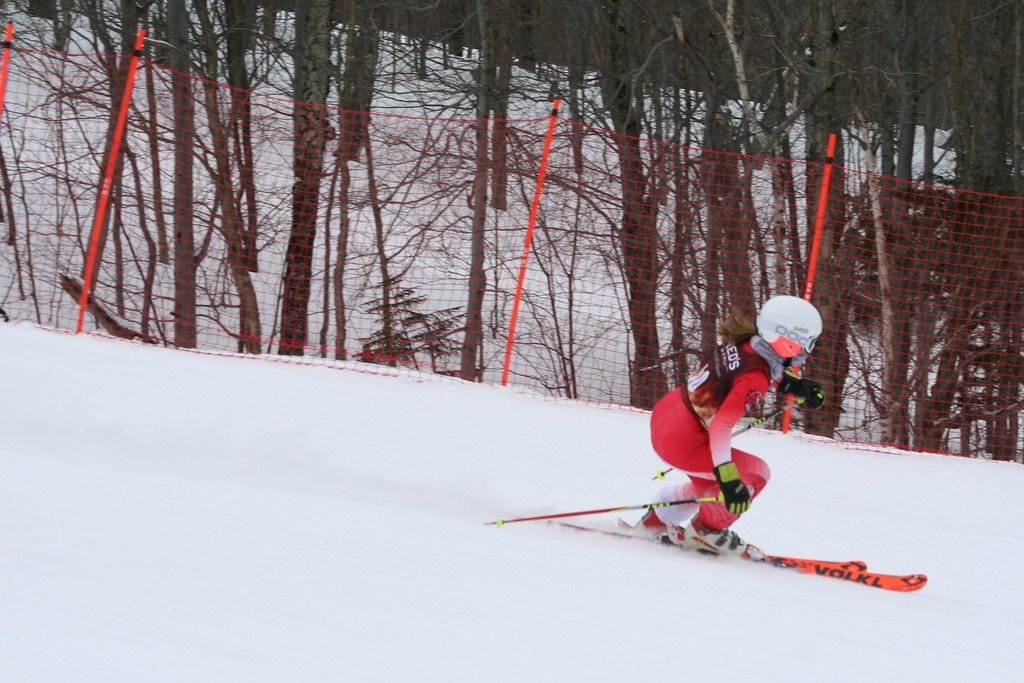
<box><xmin>755</xmin><ymin>295</ymin><xmax>822</xmax><ymax>358</ymax></box>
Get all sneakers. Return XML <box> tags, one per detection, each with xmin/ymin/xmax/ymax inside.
<box><xmin>684</xmin><ymin>522</ymin><xmax>746</xmax><ymax>555</ymax></box>
<box><xmin>633</xmin><ymin>505</ymin><xmax>667</xmax><ymax>534</ymax></box>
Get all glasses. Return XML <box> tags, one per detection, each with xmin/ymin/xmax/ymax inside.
<box><xmin>804</xmin><ymin>336</ymin><xmax>817</xmax><ymax>353</ymax></box>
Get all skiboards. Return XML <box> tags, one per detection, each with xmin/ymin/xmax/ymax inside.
<box><xmin>557</xmin><ymin>516</ymin><xmax>931</xmax><ymax>596</ymax></box>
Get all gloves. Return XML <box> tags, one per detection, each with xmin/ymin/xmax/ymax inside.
<box><xmin>778</xmin><ymin>369</ymin><xmax>825</xmax><ymax>409</ymax></box>
<box><xmin>713</xmin><ymin>461</ymin><xmax>750</xmax><ymax>513</ymax></box>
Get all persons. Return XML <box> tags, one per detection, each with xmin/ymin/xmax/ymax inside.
<box><xmin>634</xmin><ymin>296</ymin><xmax>825</xmax><ymax>553</ymax></box>
